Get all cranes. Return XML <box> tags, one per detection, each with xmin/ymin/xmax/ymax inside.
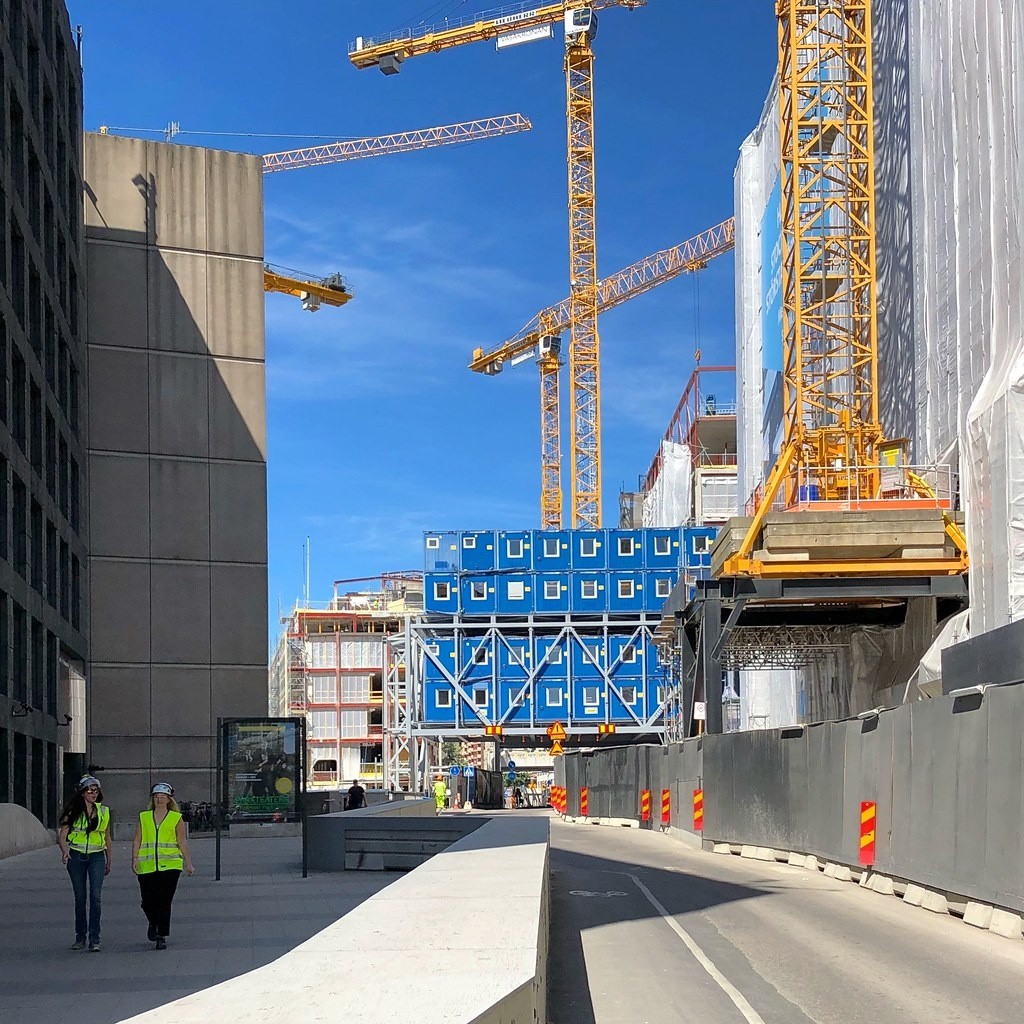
<box><xmin>348</xmin><ymin>0</ymin><xmax>650</xmax><ymax>527</ymax></box>
<box><xmin>99</xmin><ymin>112</ymin><xmax>533</xmax><ymax>176</ymax></box>
<box><xmin>262</xmin><ymin>259</ymin><xmax>354</xmax><ymax>313</ymax></box>
<box><xmin>725</xmin><ymin>0</ymin><xmax>974</xmax><ymax>579</ymax></box>
<box><xmin>467</xmin><ymin>217</ymin><xmax>737</xmax><ymax>532</ymax></box>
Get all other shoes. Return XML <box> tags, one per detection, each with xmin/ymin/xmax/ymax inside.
<box><xmin>147</xmin><ymin>924</ymin><xmax>159</xmax><ymax>941</ymax></box>
<box><xmin>156</xmin><ymin>937</ymin><xmax>167</xmax><ymax>949</ymax></box>
<box><xmin>89</xmin><ymin>943</ymin><xmax>100</xmax><ymax>952</ymax></box>
<box><xmin>72</xmin><ymin>942</ymin><xmax>86</xmax><ymax>950</ymax></box>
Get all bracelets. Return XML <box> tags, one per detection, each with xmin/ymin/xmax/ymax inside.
<box><xmin>131</xmin><ymin>856</ymin><xmax>138</xmax><ymax>861</ymax></box>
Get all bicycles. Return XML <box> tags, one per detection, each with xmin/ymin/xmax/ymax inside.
<box><xmin>177</xmin><ymin>800</ymin><xmax>215</xmax><ymax>833</ymax></box>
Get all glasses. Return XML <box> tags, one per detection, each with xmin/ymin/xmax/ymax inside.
<box><xmin>85</xmin><ymin>788</ymin><xmax>100</xmax><ymax>795</ymax></box>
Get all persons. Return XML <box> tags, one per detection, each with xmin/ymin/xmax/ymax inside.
<box><xmin>346</xmin><ymin>780</ymin><xmax>368</xmax><ymax>810</ymax></box>
<box><xmin>432</xmin><ymin>775</ymin><xmax>447</xmax><ymax>816</ymax></box>
<box><xmin>242</xmin><ymin>739</ymin><xmax>289</xmax><ymax>798</ymax></box>
<box><xmin>514</xmin><ymin>788</ymin><xmax>525</xmax><ymax>808</ymax></box>
<box><xmin>132</xmin><ymin>782</ymin><xmax>194</xmax><ymax>949</ymax></box>
<box><xmin>58</xmin><ymin>773</ymin><xmax>112</xmax><ymax>952</ymax></box>
<box><xmin>504</xmin><ymin>787</ymin><xmax>508</xmax><ymax>803</ymax></box>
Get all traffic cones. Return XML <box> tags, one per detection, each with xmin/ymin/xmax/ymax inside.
<box><xmin>453</xmin><ymin>795</ymin><xmax>460</xmax><ymax>809</ymax></box>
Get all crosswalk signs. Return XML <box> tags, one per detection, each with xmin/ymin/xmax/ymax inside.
<box><xmin>463</xmin><ymin>766</ymin><xmax>475</xmax><ymax>777</ymax></box>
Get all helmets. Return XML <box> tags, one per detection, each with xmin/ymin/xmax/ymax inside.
<box><xmin>77</xmin><ymin>775</ymin><xmax>101</xmax><ymax>790</ymax></box>
<box><xmin>150</xmin><ymin>782</ymin><xmax>173</xmax><ymax>796</ymax></box>
<box><xmin>436</xmin><ymin>774</ymin><xmax>443</xmax><ymax>781</ymax></box>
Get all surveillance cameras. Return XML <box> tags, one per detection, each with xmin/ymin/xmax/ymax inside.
<box><xmin>24</xmin><ymin>704</ymin><xmax>33</xmax><ymax>712</ymax></box>
<box><xmin>64</xmin><ymin>714</ymin><xmax>72</xmax><ymax>721</ymax></box>
